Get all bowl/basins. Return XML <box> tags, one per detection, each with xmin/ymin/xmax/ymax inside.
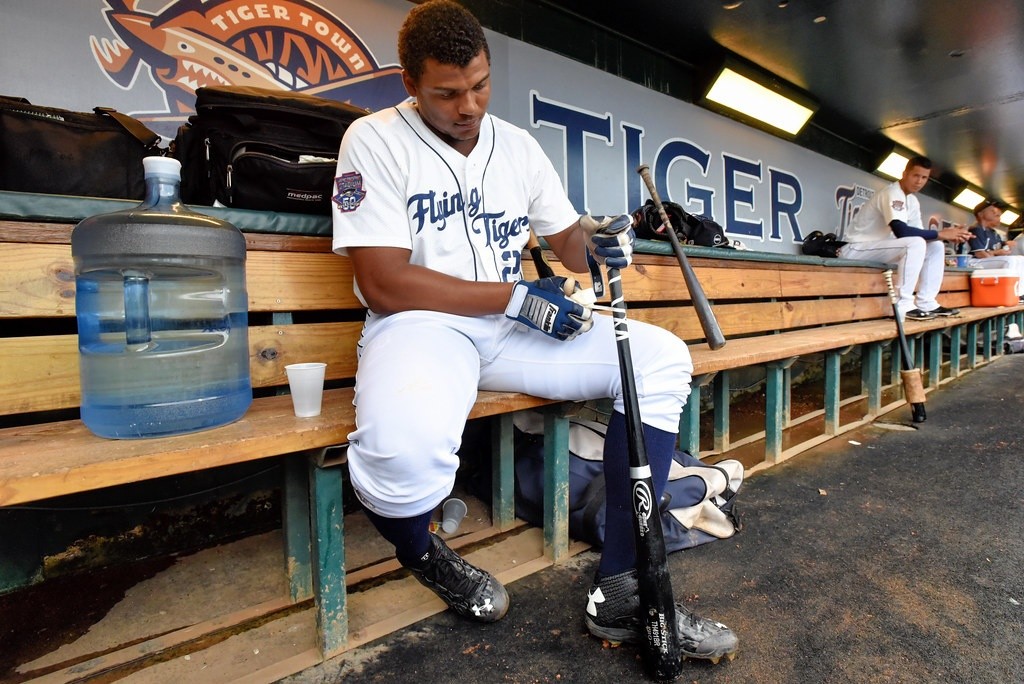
<box><xmin>945</xmin><ymin>255</ymin><xmax>974</xmax><ymax>269</ymax></box>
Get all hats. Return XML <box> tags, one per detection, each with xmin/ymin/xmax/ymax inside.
<box><xmin>691</xmin><ymin>219</ymin><xmax>736</xmax><ymax>249</ymax></box>
<box><xmin>973</xmin><ymin>201</ymin><xmax>996</xmax><ymax>216</ymax></box>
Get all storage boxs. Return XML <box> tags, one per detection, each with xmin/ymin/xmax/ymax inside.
<box><xmin>971</xmin><ymin>268</ymin><xmax>1020</xmax><ymax>307</ymax></box>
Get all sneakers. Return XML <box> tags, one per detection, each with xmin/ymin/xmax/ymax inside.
<box><xmin>905</xmin><ymin>308</ymin><xmax>937</xmax><ymax>320</ymax></box>
<box><xmin>584</xmin><ymin>568</ymin><xmax>739</xmax><ymax>664</ymax></box>
<box><xmin>929</xmin><ymin>305</ymin><xmax>961</xmax><ymax>315</ymax></box>
<box><xmin>396</xmin><ymin>531</ymin><xmax>510</xmax><ymax>621</ymax></box>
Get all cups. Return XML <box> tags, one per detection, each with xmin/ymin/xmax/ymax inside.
<box><xmin>285</xmin><ymin>362</ymin><xmax>327</xmax><ymax>417</ymax></box>
<box><xmin>441</xmin><ymin>498</ymin><xmax>467</xmax><ymax>532</ymax></box>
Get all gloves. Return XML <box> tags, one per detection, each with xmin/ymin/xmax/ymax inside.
<box><xmin>504</xmin><ymin>276</ymin><xmax>594</xmax><ymax>341</ymax></box>
<box><xmin>580</xmin><ymin>213</ymin><xmax>636</xmax><ymax>298</ymax></box>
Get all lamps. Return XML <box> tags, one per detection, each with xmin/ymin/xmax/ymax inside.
<box><xmin>949</xmin><ymin>181</ymin><xmax>987</xmax><ymax>211</ymax></box>
<box><xmin>871</xmin><ymin>145</ymin><xmax>916</xmax><ymax>181</ymax></box>
<box><xmin>999</xmin><ymin>207</ymin><xmax>1022</xmax><ymax>227</ymax></box>
<box><xmin>698</xmin><ymin>59</ymin><xmax>821</xmax><ymax>140</ymax></box>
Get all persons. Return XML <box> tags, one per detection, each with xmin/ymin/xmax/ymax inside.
<box><xmin>968</xmin><ymin>200</ymin><xmax>1024</xmax><ymax>258</ymax></box>
<box><xmin>836</xmin><ymin>154</ymin><xmax>978</xmax><ymax>321</ymax></box>
<box><xmin>332</xmin><ymin>0</ymin><xmax>740</xmax><ymax>664</ymax></box>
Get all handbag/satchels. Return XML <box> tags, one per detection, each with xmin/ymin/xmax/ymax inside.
<box><xmin>512</xmin><ymin>408</ymin><xmax>743</xmax><ymax>554</ymax></box>
<box><xmin>801</xmin><ymin>230</ymin><xmax>849</xmax><ymax>258</ymax></box>
<box><xmin>169</xmin><ymin>84</ymin><xmax>372</xmax><ymax>215</ymax></box>
<box><xmin>0</xmin><ymin>95</ymin><xmax>169</xmax><ymax>199</ymax></box>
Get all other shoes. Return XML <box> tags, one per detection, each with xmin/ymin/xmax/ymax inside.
<box><xmin>1019</xmin><ymin>295</ymin><xmax>1024</xmax><ymax>303</ymax></box>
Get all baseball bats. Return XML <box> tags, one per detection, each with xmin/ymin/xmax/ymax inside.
<box><xmin>883</xmin><ymin>269</ymin><xmax>928</xmax><ymax>423</ymax></box>
<box><xmin>605</xmin><ymin>262</ymin><xmax>684</xmax><ymax>684</ymax></box>
<box><xmin>636</xmin><ymin>164</ymin><xmax>728</xmax><ymax>350</ymax></box>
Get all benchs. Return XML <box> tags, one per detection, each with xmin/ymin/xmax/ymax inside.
<box><xmin>0</xmin><ymin>189</ymin><xmax>1024</xmax><ymax>684</ymax></box>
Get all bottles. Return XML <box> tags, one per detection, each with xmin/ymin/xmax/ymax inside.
<box><xmin>71</xmin><ymin>156</ymin><xmax>253</xmax><ymax>439</ymax></box>
